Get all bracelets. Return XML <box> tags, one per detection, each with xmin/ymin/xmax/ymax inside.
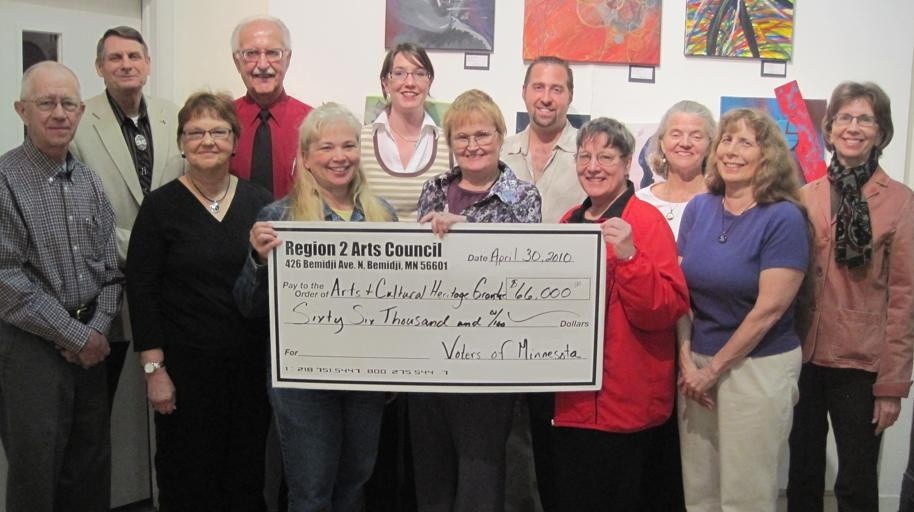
<box><xmin>618</xmin><ymin>256</ymin><xmax>633</xmax><ymax>260</ymax></box>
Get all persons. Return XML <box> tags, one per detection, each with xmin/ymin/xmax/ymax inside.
<box><xmin>408</xmin><ymin>91</ymin><xmax>542</xmax><ymax>512</ymax></box>
<box><xmin>124</xmin><ymin>91</ymin><xmax>274</xmax><ymax>510</ymax></box>
<box><xmin>360</xmin><ymin>42</ymin><xmax>458</xmax><ymax>223</ymax></box>
<box><xmin>551</xmin><ymin>117</ymin><xmax>690</xmax><ymax>509</ymax></box>
<box><xmin>1</xmin><ymin>60</ymin><xmax>123</xmax><ymax>512</ymax></box>
<box><xmin>675</xmin><ymin>108</ymin><xmax>810</xmax><ymax>512</ymax></box>
<box><xmin>789</xmin><ymin>80</ymin><xmax>913</xmax><ymax>512</ymax></box>
<box><xmin>230</xmin><ymin>11</ymin><xmax>315</xmax><ymax>199</ymax></box>
<box><xmin>234</xmin><ymin>101</ymin><xmax>398</xmax><ymax>509</ymax></box>
<box><xmin>504</xmin><ymin>56</ymin><xmax>589</xmax><ymax>225</ymax></box>
<box><xmin>70</xmin><ymin>25</ymin><xmax>193</xmax><ymax>512</ymax></box>
<box><xmin>635</xmin><ymin>100</ymin><xmax>719</xmax><ymax>242</ymax></box>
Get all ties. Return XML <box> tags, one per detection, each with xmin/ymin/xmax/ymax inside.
<box><xmin>250</xmin><ymin>109</ymin><xmax>273</xmax><ymax>194</ymax></box>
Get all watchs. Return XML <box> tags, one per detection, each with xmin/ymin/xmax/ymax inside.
<box><xmin>142</xmin><ymin>362</ymin><xmax>166</xmax><ymax>375</ymax></box>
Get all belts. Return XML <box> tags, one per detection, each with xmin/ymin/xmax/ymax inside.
<box><xmin>67</xmin><ymin>306</ymin><xmax>96</xmax><ymax>325</ymax></box>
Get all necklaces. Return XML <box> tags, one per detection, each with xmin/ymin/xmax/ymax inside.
<box><xmin>718</xmin><ymin>196</ymin><xmax>755</xmax><ymax>243</ymax></box>
<box><xmin>187</xmin><ymin>170</ymin><xmax>231</xmax><ymax>212</ymax></box>
<box><xmin>663</xmin><ymin>184</ymin><xmax>696</xmax><ymax>220</ymax></box>
<box><xmin>665</xmin><ymin>178</ymin><xmax>702</xmax><ymax>220</ymax></box>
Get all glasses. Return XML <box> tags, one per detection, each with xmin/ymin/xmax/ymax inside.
<box><xmin>181</xmin><ymin>127</ymin><xmax>233</xmax><ymax>140</ymax></box>
<box><xmin>240</xmin><ymin>47</ymin><xmax>288</xmax><ymax>63</ymax></box>
<box><xmin>451</xmin><ymin>128</ymin><xmax>499</xmax><ymax>149</ymax></box>
<box><xmin>20</xmin><ymin>96</ymin><xmax>80</xmax><ymax>112</ymax></box>
<box><xmin>389</xmin><ymin>68</ymin><xmax>429</xmax><ymax>81</ymax></box>
<box><xmin>575</xmin><ymin>149</ymin><xmax>628</xmax><ymax>167</ymax></box>
<box><xmin>832</xmin><ymin>113</ymin><xmax>879</xmax><ymax>127</ymax></box>
